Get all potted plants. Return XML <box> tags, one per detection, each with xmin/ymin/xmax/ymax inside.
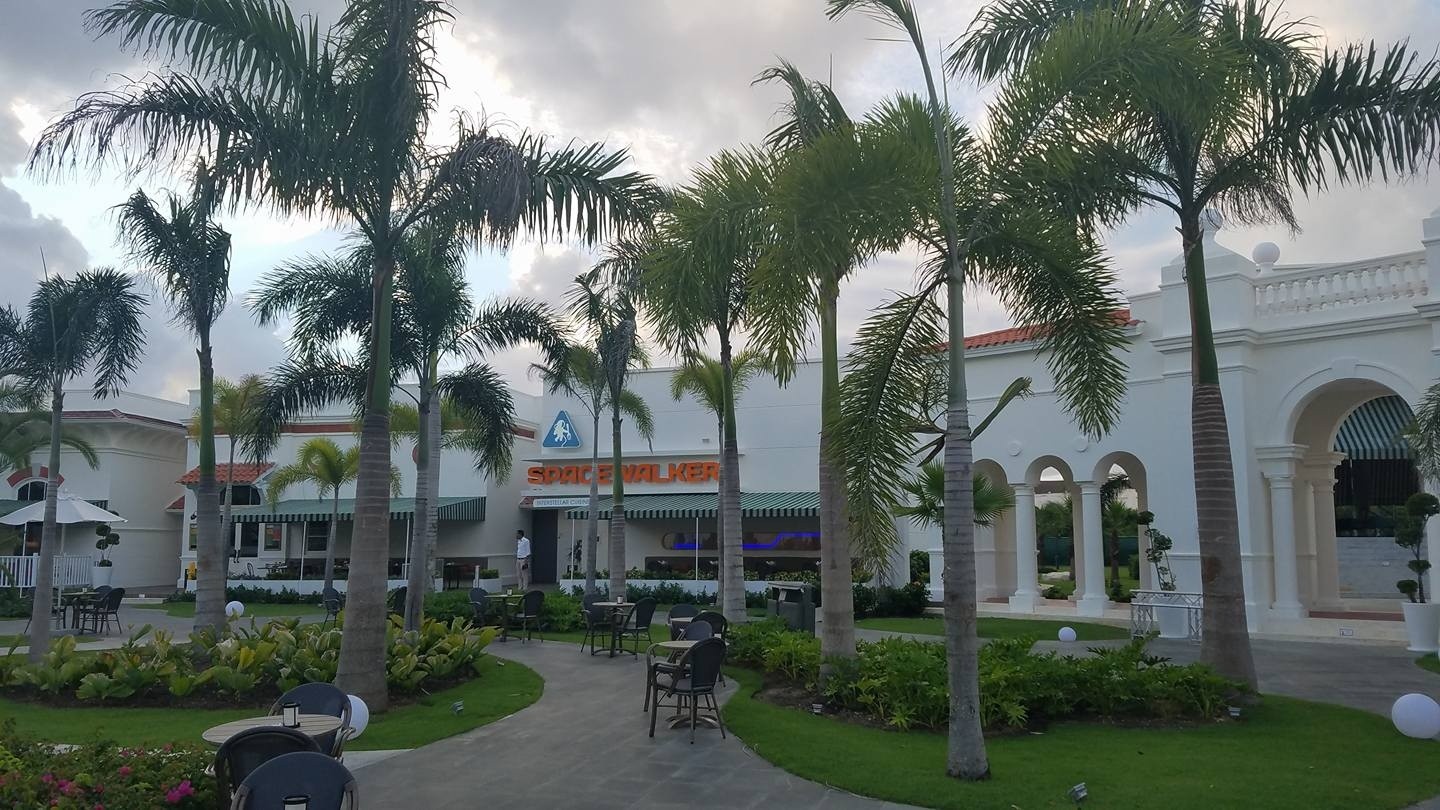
<box><xmin>1134</xmin><ymin>509</ymin><xmax>1192</xmax><ymax>641</ymax></box>
<box><xmin>92</xmin><ymin>509</ymin><xmax>120</xmax><ymax>591</ymax></box>
<box><xmin>1392</xmin><ymin>493</ymin><xmax>1439</xmax><ymax>654</ymax></box>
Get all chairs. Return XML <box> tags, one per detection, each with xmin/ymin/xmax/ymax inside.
<box><xmin>80</xmin><ymin>588</ymin><xmax>127</xmax><ymax>636</ymax></box>
<box><xmin>267</xmin><ymin>682</ymin><xmax>357</xmax><ymax>764</ymax></box>
<box><xmin>504</xmin><ymin>590</ymin><xmax>546</xmax><ymax>648</ymax></box>
<box><xmin>247</xmin><ymin>563</ymin><xmax>255</xmax><ymax>578</ymax></box>
<box><xmin>468</xmin><ymin>587</ymin><xmax>502</xmax><ymax>631</ymax></box>
<box><xmin>580</xmin><ymin>593</ymin><xmax>624</xmax><ymax>656</ymax></box>
<box><xmin>386</xmin><ymin>586</ymin><xmax>407</xmax><ymax>631</ymax></box>
<box><xmin>229</xmin><ymin>750</ymin><xmax>360</xmax><ymax>810</ymax></box>
<box><xmin>321</xmin><ymin>586</ymin><xmax>345</xmax><ymax>632</ymax></box>
<box><xmin>644</xmin><ymin>603</ymin><xmax>729</xmax><ymax>715</ymax></box>
<box><xmin>71</xmin><ymin>585</ymin><xmax>112</xmax><ymax>630</ymax></box>
<box><xmin>23</xmin><ymin>586</ymin><xmax>67</xmax><ymax>633</ymax></box>
<box><xmin>647</xmin><ymin>636</ymin><xmax>727</xmax><ymax>744</ymax></box>
<box><xmin>214</xmin><ymin>727</ymin><xmax>323</xmax><ymax>810</ymax></box>
<box><xmin>614</xmin><ymin>597</ymin><xmax>657</xmax><ymax>660</ymax></box>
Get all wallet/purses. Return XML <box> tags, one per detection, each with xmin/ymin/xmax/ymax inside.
<box><xmin>521</xmin><ymin>564</ymin><xmax>528</xmax><ymax>570</ymax></box>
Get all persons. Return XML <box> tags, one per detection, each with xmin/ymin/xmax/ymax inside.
<box><xmin>515</xmin><ymin>528</ymin><xmax>532</xmax><ymax>591</ymax></box>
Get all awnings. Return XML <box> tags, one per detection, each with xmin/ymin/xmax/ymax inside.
<box><xmin>219</xmin><ymin>495</ymin><xmax>488</xmax><ymax>527</ymax></box>
<box><xmin>564</xmin><ymin>492</ymin><xmax>821</xmax><ymax>521</ymax></box>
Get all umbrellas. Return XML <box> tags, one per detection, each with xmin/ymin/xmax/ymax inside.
<box><xmin>0</xmin><ymin>486</ymin><xmax>130</xmax><ymax>615</ymax></box>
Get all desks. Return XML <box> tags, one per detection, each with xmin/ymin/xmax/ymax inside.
<box><xmin>60</xmin><ymin>592</ymin><xmax>100</xmax><ymax>630</ymax></box>
<box><xmin>592</xmin><ymin>602</ymin><xmax>637</xmax><ymax>658</ymax></box>
<box><xmin>484</xmin><ymin>594</ymin><xmax>524</xmax><ymax>641</ymax></box>
<box><xmin>257</xmin><ymin>567</ymin><xmax>468</xmax><ymax>591</ymax></box>
<box><xmin>201</xmin><ymin>714</ymin><xmax>344</xmax><ymax>749</ymax></box>
<box><xmin>658</xmin><ymin>641</ymin><xmax>730</xmax><ymax>730</ymax></box>
